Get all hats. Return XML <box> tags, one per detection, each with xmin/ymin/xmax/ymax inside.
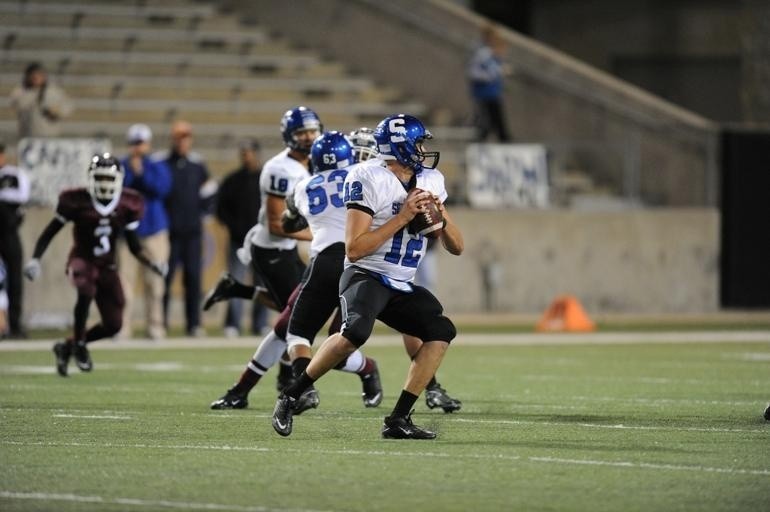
<box><xmin>126</xmin><ymin>121</ymin><xmax>260</xmax><ymax>153</ymax></box>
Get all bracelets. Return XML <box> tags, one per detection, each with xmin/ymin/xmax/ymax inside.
<box><xmin>442</xmin><ymin>214</ymin><xmax>447</xmax><ymax>229</ymax></box>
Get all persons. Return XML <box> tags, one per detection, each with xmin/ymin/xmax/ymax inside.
<box><xmin>112</xmin><ymin>107</ymin><xmax>383</xmax><ymax>408</ymax></box>
<box><xmin>1</xmin><ymin>144</ymin><xmax>33</xmax><ymax>337</ymax></box>
<box><xmin>269</xmin><ymin>114</ymin><xmax>463</xmax><ymax>441</ymax></box>
<box><xmin>8</xmin><ymin>62</ymin><xmax>73</xmax><ymax>138</ymax></box>
<box><xmin>25</xmin><ymin>150</ymin><xmax>169</xmax><ymax>382</ymax></box>
<box><xmin>465</xmin><ymin>22</ymin><xmax>516</xmax><ymax>142</ymax></box>
<box><xmin>285</xmin><ymin>131</ymin><xmax>461</xmax><ymax>414</ymax></box>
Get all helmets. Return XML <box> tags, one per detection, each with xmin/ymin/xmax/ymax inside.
<box><xmin>87</xmin><ymin>152</ymin><xmax>125</xmax><ymax>200</ymax></box>
<box><xmin>280</xmin><ymin>105</ymin><xmax>440</xmax><ymax>173</ymax></box>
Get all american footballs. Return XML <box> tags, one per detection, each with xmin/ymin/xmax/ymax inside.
<box><xmin>410</xmin><ymin>190</ymin><xmax>445</xmax><ymax>240</ymax></box>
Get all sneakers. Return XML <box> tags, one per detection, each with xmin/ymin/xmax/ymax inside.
<box><xmin>117</xmin><ymin>324</ymin><xmax>272</xmax><ymax>340</ymax></box>
<box><xmin>73</xmin><ymin>339</ymin><xmax>91</xmax><ymax>371</ymax></box>
<box><xmin>53</xmin><ymin>342</ymin><xmax>72</xmax><ymax>376</ymax></box>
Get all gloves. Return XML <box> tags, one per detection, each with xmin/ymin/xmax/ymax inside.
<box><xmin>150</xmin><ymin>260</ymin><xmax>169</xmax><ymax>276</ymax></box>
<box><xmin>23</xmin><ymin>257</ymin><xmax>40</xmax><ymax>281</ymax></box>
